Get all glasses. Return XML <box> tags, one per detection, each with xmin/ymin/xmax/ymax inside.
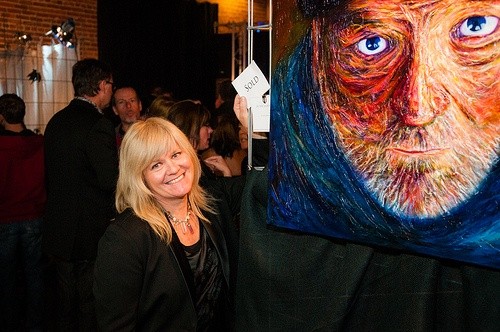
<box><xmin>106</xmin><ymin>80</ymin><xmax>116</xmax><ymax>89</ymax></box>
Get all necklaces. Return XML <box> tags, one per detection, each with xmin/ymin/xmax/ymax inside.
<box><xmin>166</xmin><ymin>199</ymin><xmax>195</xmax><ymax>234</ymax></box>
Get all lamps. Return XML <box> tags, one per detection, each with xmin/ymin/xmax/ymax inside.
<box><xmin>52</xmin><ymin>18</ymin><xmax>76</xmax><ymax>48</ymax></box>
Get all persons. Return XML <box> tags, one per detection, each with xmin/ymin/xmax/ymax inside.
<box><xmin>0</xmin><ymin>58</ymin><xmax>271</xmax><ymax>332</ymax></box>
